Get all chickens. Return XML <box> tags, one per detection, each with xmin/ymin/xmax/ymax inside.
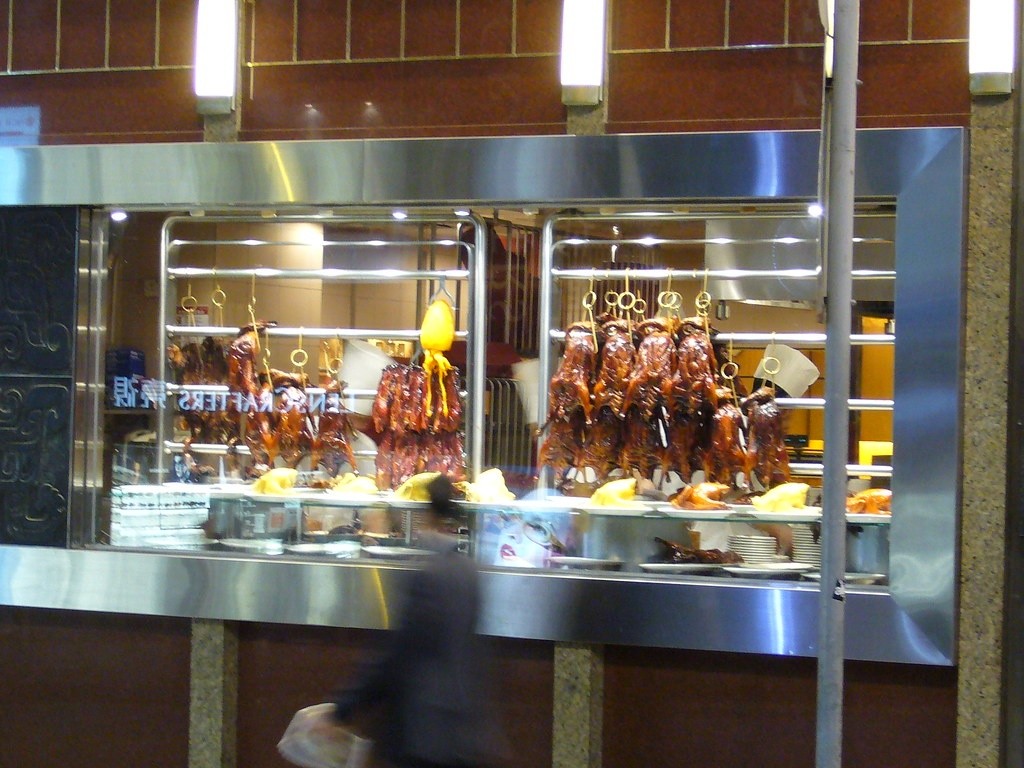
<box><xmin>169</xmin><ymin>447</ymin><xmax>892</xmax><ymax>565</ymax></box>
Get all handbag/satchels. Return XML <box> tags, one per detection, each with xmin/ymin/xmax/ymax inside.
<box><xmin>278</xmin><ymin>703</ymin><xmax>376</xmax><ymax>768</ymax></box>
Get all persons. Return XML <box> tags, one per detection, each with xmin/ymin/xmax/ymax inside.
<box><xmin>323</xmin><ymin>475</ymin><xmax>517</xmax><ymax>768</ymax></box>
<box><xmin>749</xmin><ymin>378</ymin><xmax>793</xmax><ymax>436</ymax></box>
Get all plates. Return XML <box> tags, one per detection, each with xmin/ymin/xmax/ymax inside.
<box><xmin>747</xmin><ymin>510</ymin><xmax>824</xmax><ymax>521</ymax></box>
<box><xmin>638</xmin><ymin>562</ymin><xmax>735</xmax><ymax>575</ymax></box>
<box><xmin>802</xmin><ymin>571</ymin><xmax>886</xmax><ymax>585</ymax></box>
<box><xmin>641</xmin><ymin>501</ymin><xmax>672</xmax><ymax>510</ymax></box>
<box><xmin>656</xmin><ymin>506</ymin><xmax>732</xmax><ymax>519</ymax></box>
<box><xmin>725</xmin><ymin>524</ymin><xmax>822</xmax><ymax>573</ymax></box>
<box><xmin>118</xmin><ymin>479</ymin><xmax>452</xmax><ymax>560</ymax></box>
<box><xmin>579</xmin><ymin>502</ymin><xmax>654</xmax><ymax>517</ymax></box>
<box><xmin>723</xmin><ymin>561</ymin><xmax>814</xmax><ymax>578</ymax></box>
<box><xmin>548</xmin><ymin>555</ymin><xmax>627</xmax><ymax>572</ymax></box>
<box><xmin>726</xmin><ymin>503</ymin><xmax>824</xmax><ymax>516</ymax></box>
<box><xmin>845</xmin><ymin>511</ymin><xmax>892</xmax><ymax>524</ymax></box>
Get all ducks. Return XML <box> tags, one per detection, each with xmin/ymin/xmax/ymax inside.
<box><xmin>168</xmin><ymin>320</ymin><xmax>463</xmax><ymax>494</ymax></box>
<box><xmin>531</xmin><ymin>314</ymin><xmax>794</xmax><ymax>487</ymax></box>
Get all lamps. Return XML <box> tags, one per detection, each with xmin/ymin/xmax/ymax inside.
<box><xmin>454</xmin><ymin>207</ymin><xmax>471</xmax><ymax>216</ymax></box>
<box><xmin>110</xmin><ymin>210</ymin><xmax>128</xmax><ymax>222</ymax></box>
<box><xmin>826</xmin><ymin>0</ymin><xmax>835</xmax><ymax>78</ymax></box>
<box><xmin>560</xmin><ymin>0</ymin><xmax>605</xmax><ymax>107</ymax></box>
<box><xmin>194</xmin><ymin>0</ymin><xmax>240</xmax><ymax>114</ymax></box>
<box><xmin>392</xmin><ymin>208</ymin><xmax>408</xmax><ymax>220</ymax></box>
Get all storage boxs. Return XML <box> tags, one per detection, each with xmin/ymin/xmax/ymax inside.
<box><xmin>105</xmin><ymin>348</ymin><xmax>146</xmax><ymax>408</ymax></box>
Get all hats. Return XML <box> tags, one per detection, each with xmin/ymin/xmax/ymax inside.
<box><xmin>753</xmin><ymin>344</ymin><xmax>820</xmax><ymax>398</ymax></box>
<box><xmin>338</xmin><ymin>337</ymin><xmax>398</xmax><ymax>417</ymax></box>
<box><xmin>510</xmin><ymin>358</ymin><xmax>561</xmax><ymax>424</ymax></box>
